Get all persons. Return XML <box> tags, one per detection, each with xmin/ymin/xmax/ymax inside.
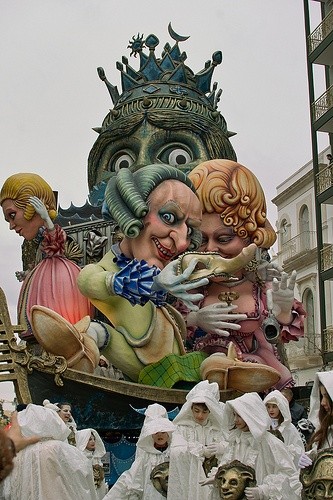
<box><xmin>29</xmin><ymin>165</ymin><xmax>283</xmax><ymax>395</ymax></box>
<box><xmin>0</xmin><ymin>173</ymin><xmax>95</xmax><ymax>341</ymax></box>
<box><xmin>170</xmin><ymin>158</ymin><xmax>308</xmax><ymax>396</ymax></box>
<box><xmin>0</xmin><ymin>398</ymin><xmax>108</xmax><ymax>500</ymax></box>
<box><xmin>298</xmin><ymin>371</ymin><xmax>333</xmax><ymax>500</ymax></box>
<box><xmin>102</xmin><ymin>380</ymin><xmax>305</xmax><ymax>500</ymax></box>
